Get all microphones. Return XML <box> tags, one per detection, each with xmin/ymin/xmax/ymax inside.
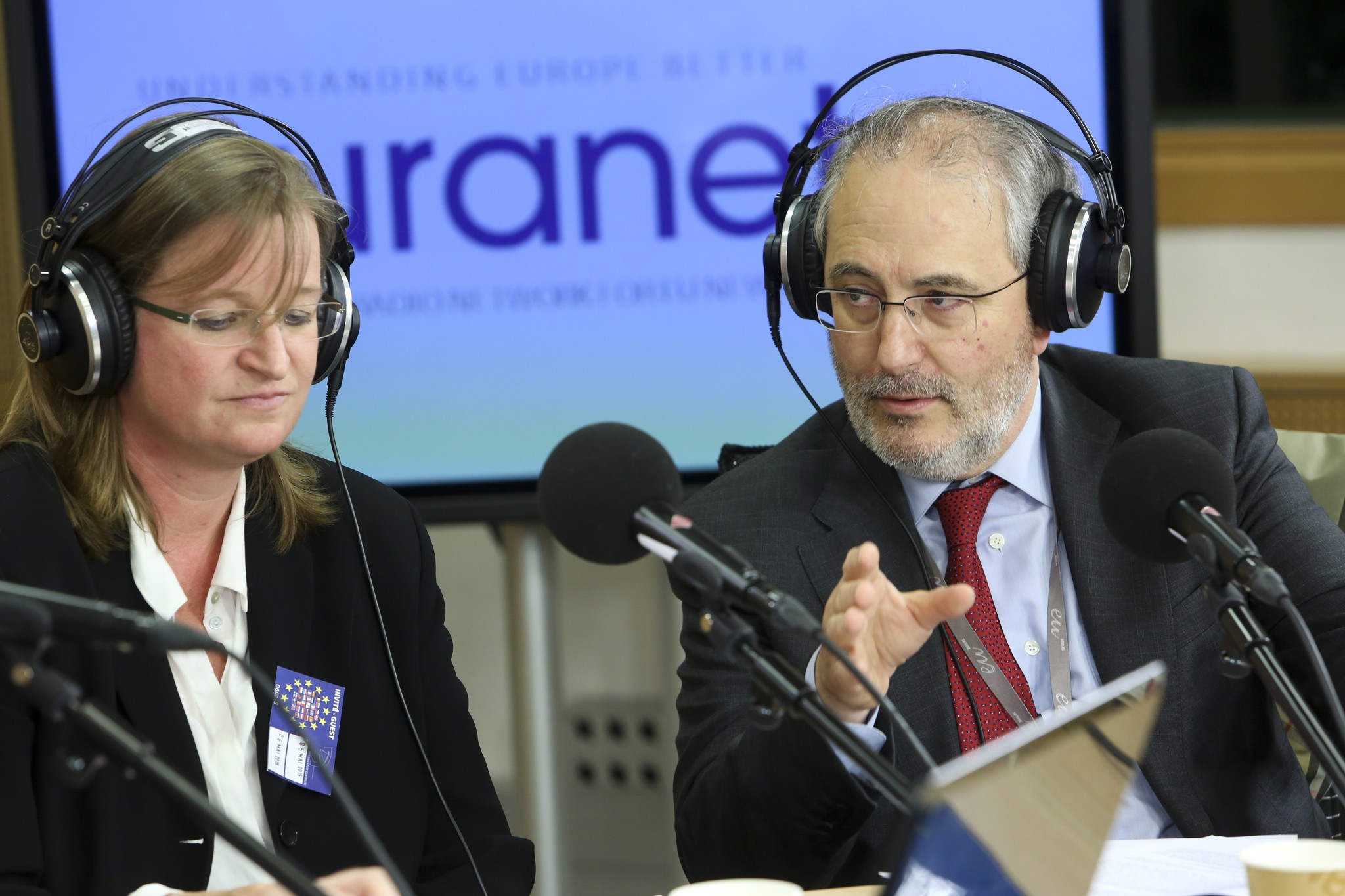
<box><xmin>537</xmin><ymin>421</ymin><xmax>831</xmax><ymax>637</ymax></box>
<box><xmin>1095</xmin><ymin>429</ymin><xmax>1291</xmax><ymax>608</ymax></box>
<box><xmin>1</xmin><ymin>581</ymin><xmax>217</xmax><ymax>655</ymax></box>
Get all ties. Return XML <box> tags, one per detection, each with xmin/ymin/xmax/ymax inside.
<box><xmin>933</xmin><ymin>476</ymin><xmax>1041</xmax><ymax>752</ymax></box>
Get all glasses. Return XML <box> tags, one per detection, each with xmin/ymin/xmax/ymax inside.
<box><xmin>127</xmin><ymin>295</ymin><xmax>346</xmax><ymax>346</ymax></box>
<box><xmin>811</xmin><ymin>271</ymin><xmax>1033</xmax><ymax>338</ymax></box>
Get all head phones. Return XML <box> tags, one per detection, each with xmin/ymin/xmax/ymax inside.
<box><xmin>14</xmin><ymin>94</ymin><xmax>359</xmax><ymax>394</ymax></box>
<box><xmin>768</xmin><ymin>49</ymin><xmax>1128</xmax><ymax>333</ymax></box>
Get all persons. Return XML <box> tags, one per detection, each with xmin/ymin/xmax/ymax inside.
<box><xmin>0</xmin><ymin>115</ymin><xmax>538</xmax><ymax>896</ymax></box>
<box><xmin>666</xmin><ymin>98</ymin><xmax>1345</xmax><ymax>890</ymax></box>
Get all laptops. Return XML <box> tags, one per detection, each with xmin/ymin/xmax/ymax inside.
<box><xmin>887</xmin><ymin>653</ymin><xmax>1166</xmax><ymax>895</ymax></box>
